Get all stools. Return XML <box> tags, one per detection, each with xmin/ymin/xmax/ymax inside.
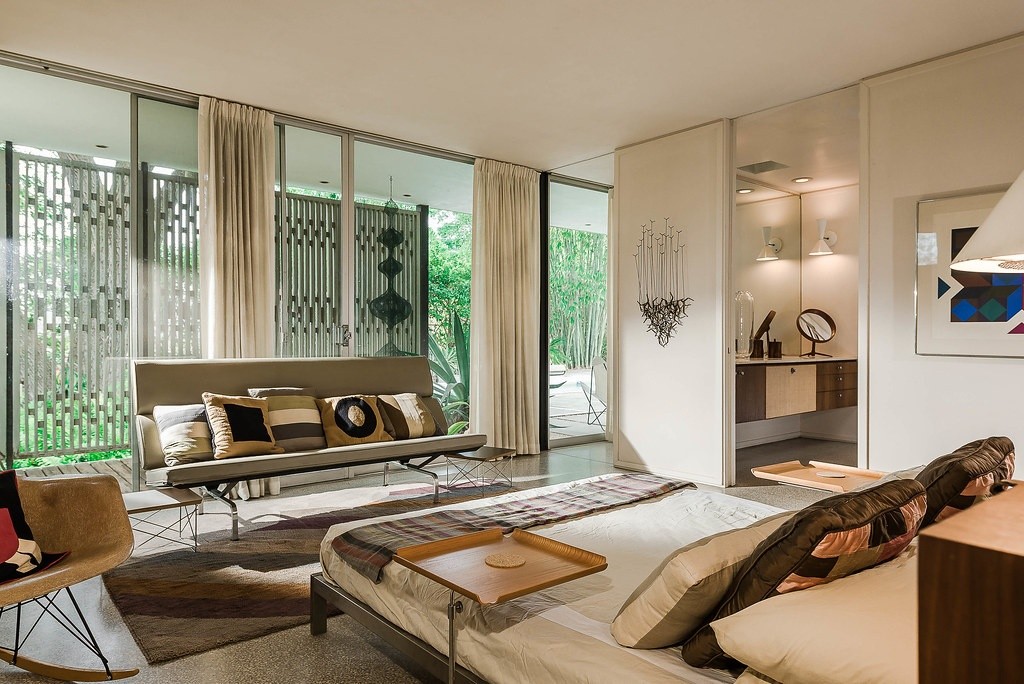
<box><xmin>122</xmin><ymin>484</ymin><xmax>203</xmax><ymax>555</ymax></box>
<box><xmin>442</xmin><ymin>445</ymin><xmax>517</xmax><ymax>498</ymax></box>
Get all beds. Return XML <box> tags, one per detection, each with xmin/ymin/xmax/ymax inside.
<box><xmin>308</xmin><ymin>473</ymin><xmax>1024</xmax><ymax>684</ymax></box>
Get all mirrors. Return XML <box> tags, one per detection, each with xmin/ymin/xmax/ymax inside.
<box><xmin>798</xmin><ymin>308</ymin><xmax>837</xmax><ymax>358</ymax></box>
<box><xmin>734</xmin><ymin>175</ymin><xmax>803</xmax><ymax>356</ymax></box>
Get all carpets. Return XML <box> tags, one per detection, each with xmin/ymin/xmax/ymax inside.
<box><xmin>102</xmin><ymin>478</ymin><xmax>525</xmax><ymax>664</ymax></box>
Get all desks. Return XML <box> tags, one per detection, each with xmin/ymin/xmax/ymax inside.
<box><xmin>549</xmin><ymin>373</ymin><xmax>568</xmax><ymax>390</ymax></box>
<box><xmin>393</xmin><ymin>526</ymin><xmax>610</xmax><ymax>684</ymax></box>
<box><xmin>550</xmin><ymin>363</ymin><xmax>566</xmax><ymax>372</ymax></box>
<box><xmin>749</xmin><ymin>457</ymin><xmax>888</xmax><ymax>490</ymax></box>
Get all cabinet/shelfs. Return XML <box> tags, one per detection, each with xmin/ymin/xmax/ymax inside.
<box><xmin>736</xmin><ymin>362</ymin><xmax>858</xmax><ymax>428</ymax></box>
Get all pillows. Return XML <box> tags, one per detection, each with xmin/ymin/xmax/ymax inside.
<box><xmin>315</xmin><ymin>393</ymin><xmax>388</xmax><ymax>450</ymax></box>
<box><xmin>379</xmin><ymin>393</ymin><xmax>436</xmax><ymax>442</ymax></box>
<box><xmin>682</xmin><ymin>478</ymin><xmax>928</xmax><ymax>670</ymax></box>
<box><xmin>202</xmin><ymin>392</ymin><xmax>285</xmax><ymax>459</ymax></box>
<box><xmin>850</xmin><ymin>462</ymin><xmax>926</xmax><ymax>492</ymax></box>
<box><xmin>248</xmin><ymin>385</ymin><xmax>327</xmax><ymax>451</ymax></box>
<box><xmin>0</xmin><ymin>470</ymin><xmax>43</xmax><ymax>580</ymax></box>
<box><xmin>609</xmin><ymin>510</ymin><xmax>796</xmax><ymax>650</ymax></box>
<box><xmin>712</xmin><ymin>541</ymin><xmax>917</xmax><ymax>684</ymax></box>
<box><xmin>913</xmin><ymin>436</ymin><xmax>1016</xmax><ymax>532</ymax></box>
<box><xmin>154</xmin><ymin>403</ymin><xmax>212</xmax><ymax>464</ymax></box>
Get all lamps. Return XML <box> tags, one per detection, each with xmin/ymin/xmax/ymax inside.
<box><xmin>756</xmin><ymin>221</ymin><xmax>784</xmax><ymax>261</ymax></box>
<box><xmin>808</xmin><ymin>216</ymin><xmax>838</xmax><ymax>255</ymax></box>
<box><xmin>949</xmin><ymin>170</ymin><xmax>1024</xmax><ymax>273</ymax></box>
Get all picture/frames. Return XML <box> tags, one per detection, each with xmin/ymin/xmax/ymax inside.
<box><xmin>910</xmin><ymin>193</ymin><xmax>1024</xmax><ymax>359</ymax></box>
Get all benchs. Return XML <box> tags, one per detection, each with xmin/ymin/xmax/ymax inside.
<box><xmin>127</xmin><ymin>353</ymin><xmax>491</xmax><ymax>544</ymax></box>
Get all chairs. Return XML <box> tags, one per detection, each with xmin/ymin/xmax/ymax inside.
<box><xmin>578</xmin><ymin>354</ymin><xmax>608</xmax><ymax>431</ymax></box>
<box><xmin>0</xmin><ymin>465</ymin><xmax>140</xmax><ymax>683</ymax></box>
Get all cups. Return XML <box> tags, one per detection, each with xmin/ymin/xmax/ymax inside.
<box><xmin>768</xmin><ymin>341</ymin><xmax>782</xmax><ymax>360</ymax></box>
<box><xmin>749</xmin><ymin>340</ymin><xmax>763</xmax><ymax>358</ymax></box>
